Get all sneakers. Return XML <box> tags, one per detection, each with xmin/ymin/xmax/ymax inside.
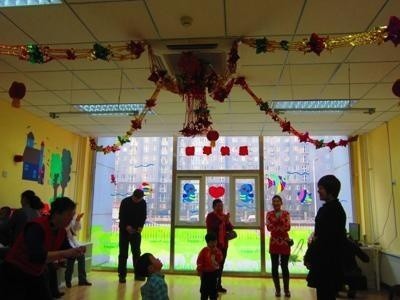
<box><xmin>218</xmin><ymin>288</ymin><xmax>226</xmax><ymax>292</ymax></box>
<box><xmin>65</xmin><ymin>280</ymin><xmax>72</xmax><ymax>287</ymax></box>
<box><xmin>79</xmin><ymin>281</ymin><xmax>92</xmax><ymax>285</ymax></box>
<box><xmin>134</xmin><ymin>276</ymin><xmax>145</xmax><ymax>280</ymax></box>
<box><xmin>284</xmin><ymin>288</ymin><xmax>290</xmax><ymax>296</ymax></box>
<box><xmin>119</xmin><ymin>276</ymin><xmax>126</xmax><ymax>282</ymax></box>
<box><xmin>275</xmin><ymin>289</ymin><xmax>281</xmax><ymax>296</ymax></box>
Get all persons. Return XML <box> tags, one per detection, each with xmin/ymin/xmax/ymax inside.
<box><xmin>138</xmin><ymin>253</ymin><xmax>169</xmax><ymax>300</ymax></box>
<box><xmin>0</xmin><ymin>190</ymin><xmax>92</xmax><ymax>300</ymax></box>
<box><xmin>118</xmin><ymin>189</ymin><xmax>145</xmax><ymax>283</ymax></box>
<box><xmin>304</xmin><ymin>175</ymin><xmax>346</xmax><ymax>300</ymax></box>
<box><xmin>197</xmin><ymin>232</ymin><xmax>223</xmax><ymax>300</ymax></box>
<box><xmin>266</xmin><ymin>195</ymin><xmax>290</xmax><ymax>297</ymax></box>
<box><xmin>206</xmin><ymin>199</ymin><xmax>237</xmax><ymax>296</ymax></box>
<box><xmin>335</xmin><ymin>229</ymin><xmax>370</xmax><ymax>298</ymax></box>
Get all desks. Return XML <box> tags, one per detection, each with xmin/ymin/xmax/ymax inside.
<box><xmin>354</xmin><ymin>245</ymin><xmax>382</xmax><ymax>292</ymax></box>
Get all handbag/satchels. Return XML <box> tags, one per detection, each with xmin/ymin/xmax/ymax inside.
<box><xmin>5</xmin><ymin>237</ymin><xmax>46</xmax><ymax>276</ymax></box>
<box><xmin>225</xmin><ymin>231</ymin><xmax>236</xmax><ymax>241</ymax></box>
<box><xmin>287</xmin><ymin>239</ymin><xmax>293</xmax><ymax>246</ymax></box>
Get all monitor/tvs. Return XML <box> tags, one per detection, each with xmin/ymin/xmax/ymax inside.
<box><xmin>349</xmin><ymin>223</ymin><xmax>360</xmax><ymax>241</ymax></box>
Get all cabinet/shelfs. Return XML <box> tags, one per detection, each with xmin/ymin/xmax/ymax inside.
<box><xmin>56</xmin><ymin>242</ymin><xmax>93</xmax><ymax>286</ymax></box>
